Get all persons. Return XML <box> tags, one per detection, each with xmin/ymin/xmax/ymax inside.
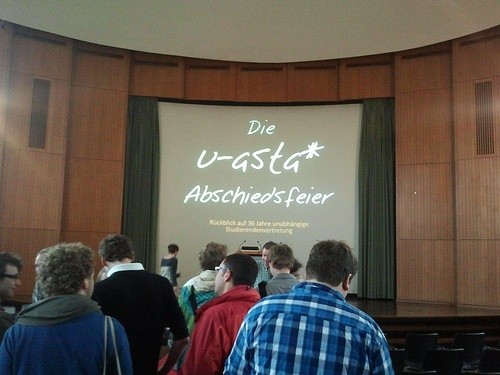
<box><xmin>160</xmin><ymin>244</ymin><xmax>181</xmax><ymax>295</ymax></box>
<box><xmin>253</xmin><ymin>243</ymin><xmax>301</xmax><ymax>299</ymax></box>
<box><xmin>89</xmin><ymin>234</ymin><xmax>190</xmax><ymax>375</ymax></box>
<box><xmin>0</xmin><ymin>253</ymin><xmax>24</xmax><ymax>344</ymax></box>
<box><xmin>224</xmin><ymin>240</ymin><xmax>394</xmax><ymax>375</ymax></box>
<box><xmin>261</xmin><ymin>240</ymin><xmax>277</xmax><ymax>281</ymax></box>
<box><xmin>180</xmin><ymin>254</ymin><xmax>261</xmax><ymax>375</ymax></box>
<box><xmin>175</xmin><ymin>242</ymin><xmax>230</xmax><ymax>375</ymax></box>
<box><xmin>177</xmin><ymin>242</ymin><xmax>227</xmax><ymax>370</ymax></box>
<box><xmin>0</xmin><ymin>242</ymin><xmax>133</xmax><ymax>375</ymax></box>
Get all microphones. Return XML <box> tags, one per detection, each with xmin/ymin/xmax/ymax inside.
<box><xmin>257</xmin><ymin>241</ymin><xmax>261</xmax><ymax>247</ymax></box>
<box><xmin>239</xmin><ymin>241</ymin><xmax>246</xmax><ymax>250</ymax></box>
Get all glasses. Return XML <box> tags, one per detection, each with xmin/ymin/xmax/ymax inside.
<box><xmin>215</xmin><ymin>266</ymin><xmax>233</xmax><ymax>278</ymax></box>
<box><xmin>1</xmin><ymin>273</ymin><xmax>19</xmax><ymax>280</ymax></box>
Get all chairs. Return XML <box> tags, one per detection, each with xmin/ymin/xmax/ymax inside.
<box><xmin>384</xmin><ymin>331</ymin><xmax>500</xmax><ymax>375</ymax></box>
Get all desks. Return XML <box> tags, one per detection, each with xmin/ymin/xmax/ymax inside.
<box><xmin>246</xmin><ymin>256</ymin><xmax>273</xmax><ymax>289</ymax></box>
